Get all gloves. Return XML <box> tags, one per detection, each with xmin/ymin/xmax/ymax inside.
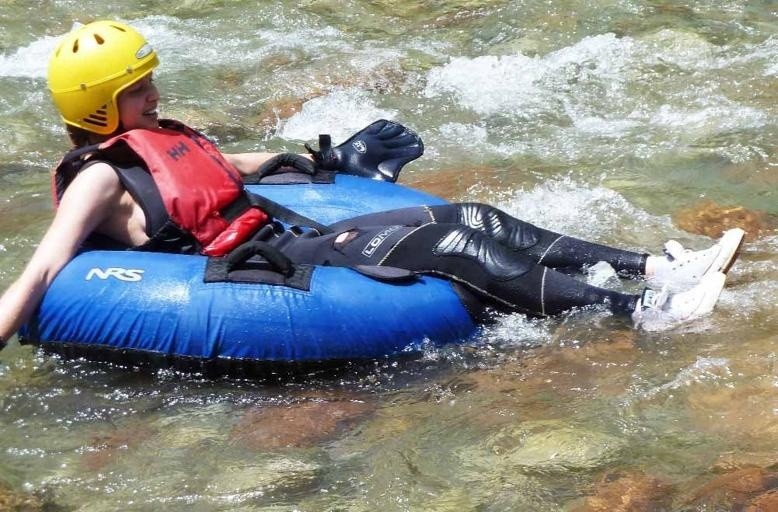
<box><xmin>334</xmin><ymin>119</ymin><xmax>424</xmax><ymax>182</ymax></box>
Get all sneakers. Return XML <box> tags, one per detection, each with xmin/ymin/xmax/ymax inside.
<box><xmin>663</xmin><ymin>228</ymin><xmax>746</xmax><ymax>294</ymax></box>
<box><xmin>631</xmin><ymin>272</ymin><xmax>727</xmax><ymax>332</ymax></box>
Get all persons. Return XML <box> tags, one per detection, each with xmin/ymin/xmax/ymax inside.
<box><xmin>0</xmin><ymin>18</ymin><xmax>747</xmax><ymax>357</ymax></box>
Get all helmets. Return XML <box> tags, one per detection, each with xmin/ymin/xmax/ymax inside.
<box><xmin>47</xmin><ymin>21</ymin><xmax>160</xmax><ymax>135</ymax></box>
